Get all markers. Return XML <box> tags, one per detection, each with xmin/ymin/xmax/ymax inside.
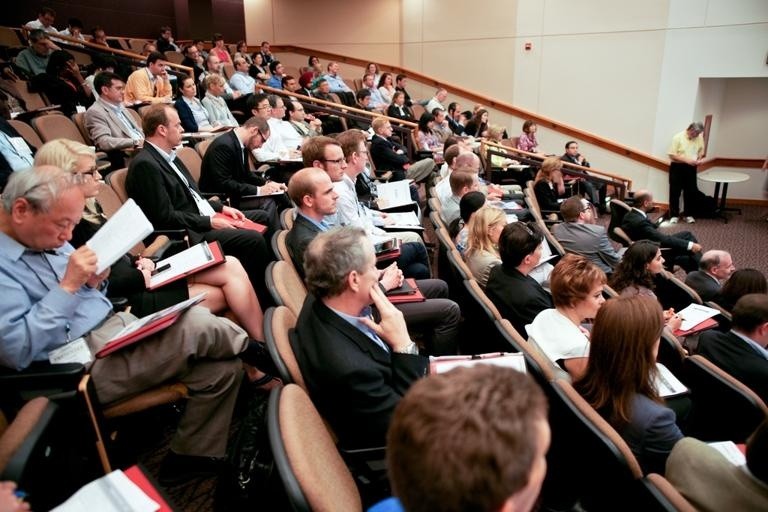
<box><xmin>147</xmin><ymin>264</ymin><xmax>171</xmax><ymax>276</ymax></box>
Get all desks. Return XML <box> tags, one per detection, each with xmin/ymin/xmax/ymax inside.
<box><xmin>697</xmin><ymin>170</ymin><xmax>750</xmax><ymax>221</ymax></box>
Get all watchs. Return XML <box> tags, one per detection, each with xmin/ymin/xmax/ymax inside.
<box><xmin>395</xmin><ymin>344</ymin><xmax>421</xmax><ymax>356</ymax></box>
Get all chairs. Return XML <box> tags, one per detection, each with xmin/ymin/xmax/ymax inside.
<box><xmin>501</xmin><ymin>138</ymin><xmax>520</xmax><ymax>159</ymax></box>
<box><xmin>607</xmin><ymin>198</ymin><xmax>632</xmax><ymax>239</ymax></box>
<box><xmin>342</xmin><ymin>79</ymin><xmax>356</xmax><ymax>93</ymax></box>
<box><xmin>705</xmin><ymin>299</ymin><xmax>731</xmax><ymax>328</ymax></box>
<box><xmin>263</xmin><ymin>304</ymin><xmax>337</xmax><ymax>442</ymax></box>
<box><xmin>280</xmin><ymin>208</ymin><xmax>296</xmax><ymax>230</ymax></box>
<box><xmin>105</xmin><ymin>167</ymin><xmax>129</xmax><ymax>204</ymax></box>
<box><xmin>228</xmin><ymin>43</ymin><xmax>240</xmax><ymax>55</ymax></box>
<box><xmin>411</xmin><ymin>105</ymin><xmax>425</xmax><ymax>121</ymax></box>
<box><xmin>270</xmin><ymin>230</ymin><xmax>310</xmax><ymax>293</ymax></box>
<box><xmin>0</xmin><ymin>361</ymin><xmax>189</xmax><ymax>473</ymax></box>
<box><xmin>31</xmin><ymin>114</ymin><xmax>112</xmax><ymax>172</ymax></box>
<box><xmin>658</xmin><ymin>330</ymin><xmax>688</xmax><ymax>379</ymax></box>
<box><xmin>446</xmin><ymin>247</ymin><xmax>472</xmax><ymax>279</ymax></box>
<box><xmin>355</xmin><ymin>78</ymin><xmax>363</xmax><ymax>91</ymax></box>
<box><xmin>686</xmin><ymin>354</ymin><xmax>767</xmax><ymax>443</ymax></box>
<box><xmin>9</xmin><ymin>80</ymin><xmax>62</xmax><ymax>115</ymax></box>
<box><xmin>613</xmin><ymin>227</ymin><xmax>633</xmax><ymax>250</ymax></box>
<box><xmin>164</xmin><ymin>51</ymin><xmax>185</xmax><ymax>65</ymax></box>
<box><xmin>429</xmin><ymin>186</ymin><xmax>437</xmax><ymax>195</ymax></box>
<box><xmin>129</xmin><ymin>39</ymin><xmax>149</xmax><ymax>54</ymax></box>
<box><xmin>524</xmin><ymin>196</ymin><xmax>533</xmax><ymax>208</ymax></box>
<box><xmin>512</xmin><ymin>137</ymin><xmax>520</xmax><ymax>146</ymax></box>
<box><xmin>463</xmin><ymin>278</ymin><xmax>501</xmax><ymax>318</ymax></box>
<box><xmin>223</xmin><ymin>64</ymin><xmax>235</xmax><ymax>78</ymax></box>
<box><xmin>529</xmin><ymin>207</ymin><xmax>542</xmax><ymax>220</ymax></box>
<box><xmin>523</xmin><ymin>186</ymin><xmax>563</xmax><ymax>225</ymax></box>
<box><xmin>538</xmin><ymin>219</ymin><xmax>550</xmax><ymax>234</ymax></box>
<box><xmin>430</xmin><ymin>210</ymin><xmax>445</xmax><ymax>227</ymax></box>
<box><xmin>266</xmin><ymin>383</ymin><xmax>363</xmax><ymax>512</ymax></box>
<box><xmin>195</xmin><ymin>139</ymin><xmax>211</xmax><ymax>153</ymax></box>
<box><xmin>71</xmin><ymin>112</ymin><xmax>96</xmax><ymax>144</ymax></box>
<box><xmin>429</xmin><ymin>197</ymin><xmax>442</xmax><ymax>211</ymax></box>
<box><xmin>263</xmin><ymin>259</ymin><xmax>308</xmax><ymax>319</ymax></box>
<box><xmin>124</xmin><ymin>107</ymin><xmax>144</xmax><ymax>128</ymax></box>
<box><xmin>666</xmin><ymin>276</ymin><xmax>702</xmax><ymax>313</ymax></box>
<box><xmin>7</xmin><ymin>119</ymin><xmax>44</xmax><ymax>151</ymax></box>
<box><xmin>641</xmin><ymin>472</ymin><xmax>702</xmax><ymax>512</ymax></box>
<box><xmin>410</xmin><ymin>129</ymin><xmax>432</xmax><ymax>158</ymax></box>
<box><xmin>495</xmin><ymin>316</ymin><xmax>557</xmax><ymax>382</ymax></box>
<box><xmin>360</xmin><ymin>150</ymin><xmax>427</xmax><ymax>209</ymax></box>
<box><xmin>175</xmin><ymin>147</ymin><xmax>231</xmax><ymax>205</ymax></box>
<box><xmin>93</xmin><ymin>185</ymin><xmax>147</xmax><ymax>261</ymax></box>
<box><xmin>116</xmin><ymin>38</ymin><xmax>129</xmax><ymax>49</ymax></box>
<box><xmin>548</xmin><ymin>233</ymin><xmax>564</xmax><ymax>257</ymax></box>
<box><xmin>549</xmin><ymin>379</ymin><xmax>643</xmax><ymax>512</ymax></box>
<box><xmin>527</xmin><ymin>180</ymin><xmax>563</xmax><ymax>215</ymax></box>
<box><xmin>138</xmin><ymin>105</ymin><xmax>151</xmax><ymax>116</ymax></box>
<box><xmin>435</xmin><ymin>227</ymin><xmax>456</xmax><ymax>251</ymax></box>
<box><xmin>602</xmin><ymin>284</ymin><xmax>617</xmax><ymax>302</ymax></box>
<box><xmin>434</xmin><ymin>175</ymin><xmax>443</xmax><ymax>183</ymax></box>
<box><xmin>300</xmin><ymin>67</ymin><xmax>310</xmax><ymax>75</ymax></box>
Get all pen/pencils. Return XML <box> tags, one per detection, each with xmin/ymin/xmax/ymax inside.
<box><xmin>265</xmin><ymin>175</ymin><xmax>269</xmax><ymax>183</ymax></box>
<box><xmin>61</xmin><ymin>251</ymin><xmax>71</xmax><ymax>256</ymax></box>
<box><xmin>14</xmin><ymin>490</ymin><xmax>29</xmax><ymax>498</ymax></box>
<box><xmin>676</xmin><ymin>316</ymin><xmax>686</xmax><ymax>321</ymax></box>
<box><xmin>434</xmin><ymin>351</ymin><xmax>505</xmax><ymax>362</ymax></box>
<box><xmin>137</xmin><ymin>255</ymin><xmax>160</xmax><ymax>260</ymax></box>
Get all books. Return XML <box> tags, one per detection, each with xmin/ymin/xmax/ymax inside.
<box><xmin>87</xmin><ymin>198</ymin><xmax>154</xmax><ymax>276</ymax></box>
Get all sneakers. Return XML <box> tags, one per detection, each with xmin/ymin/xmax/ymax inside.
<box><xmin>669</xmin><ymin>214</ymin><xmax>680</xmax><ymax>226</ymax></box>
<box><xmin>684</xmin><ymin>214</ymin><xmax>696</xmax><ymax>224</ymax></box>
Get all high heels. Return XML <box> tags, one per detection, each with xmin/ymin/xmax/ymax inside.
<box><xmin>246</xmin><ymin>371</ymin><xmax>282</xmax><ymax>390</ymax></box>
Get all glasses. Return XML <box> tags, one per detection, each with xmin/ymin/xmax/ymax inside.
<box><xmin>321</xmin><ymin>157</ymin><xmax>348</xmax><ymax>164</ymax></box>
<box><xmin>255</xmin><ymin>126</ymin><xmax>268</xmax><ymax>143</ymax></box>
<box><xmin>66</xmin><ymin>165</ymin><xmax>101</xmax><ymax>181</ymax></box>
<box><xmin>255</xmin><ymin>104</ymin><xmax>275</xmax><ymax>112</ymax></box>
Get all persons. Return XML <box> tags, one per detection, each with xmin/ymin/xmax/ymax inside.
<box><xmin>479</xmin><ymin>125</ymin><xmax>538</xmax><ymax>190</ymax></box>
<box><xmin>124</xmin><ymin>52</ymin><xmax>173</xmax><ymax>104</ymax></box>
<box><xmin>336</xmin><ymin>130</ymin><xmax>434</xmax><ymax>279</ymax></box>
<box><xmin>201</xmin><ymin>76</ymin><xmax>239</xmax><ymax>128</ymax></box>
<box><xmin>432</xmin><ymin>108</ymin><xmax>476</xmax><ymax>145</ymax></box>
<box><xmin>465</xmin><ymin>109</ymin><xmax>489</xmax><ymax>143</ymax></box>
<box><xmin>445</xmin><ymin>145</ymin><xmax>524</xmax><ymax>208</ymax></box>
<box><xmin>0</xmin><ymin>478</ymin><xmax>33</xmax><ymax>512</ymax></box>
<box><xmin>229</xmin><ymin>57</ymin><xmax>266</xmax><ymax>107</ymax></box>
<box><xmin>16</xmin><ymin>30</ymin><xmax>63</xmax><ymax>78</ymax></box>
<box><xmin>209</xmin><ymin>33</ymin><xmax>236</xmax><ymax>75</ymax></box>
<box><xmin>368</xmin><ymin>366</ymin><xmax>550</xmax><ymax>512</ymax></box>
<box><xmin>460</xmin><ymin>136</ymin><xmax>475</xmax><ymax>148</ymax></box>
<box><xmin>443</xmin><ymin>136</ymin><xmax>524</xmax><ymax>195</ymax></box>
<box><xmin>295</xmin><ymin>226</ymin><xmax>431</xmax><ymax>494</ymax></box>
<box><xmin>709</xmin><ymin>268</ymin><xmax>768</xmax><ymax>313</ymax></box>
<box><xmin>365</xmin><ymin>62</ymin><xmax>382</xmax><ymax>86</ymax></box>
<box><xmin>0</xmin><ymin>166</ymin><xmax>280</xmax><ymax>487</ymax></box>
<box><xmin>295</xmin><ymin>73</ymin><xmax>343</xmax><ymax>135</ymax></box>
<box><xmin>281</xmin><ymin>76</ymin><xmax>330</xmax><ymax>122</ymax></box>
<box><xmin>395</xmin><ymin>75</ymin><xmax>433</xmax><ymax>106</ymax></box>
<box><xmin>233</xmin><ymin>41</ymin><xmax>253</xmax><ymax>66</ymax></box>
<box><xmin>434</xmin><ymin>151</ymin><xmax>537</xmax><ymax>222</ymax></box>
<box><xmin>24</xmin><ymin>8</ymin><xmax>60</xmax><ymax>47</ymax></box>
<box><xmin>534</xmin><ymin>156</ymin><xmax>598</xmax><ymax>225</ymax></box>
<box><xmin>60</xmin><ymin>18</ymin><xmax>86</xmax><ymax>49</ymax></box>
<box><xmin>0</xmin><ymin>59</ymin><xmax>61</xmax><ymax>120</ymax></box>
<box><xmin>0</xmin><ymin>92</ymin><xmax>38</xmax><ymax>195</ymax></box>
<box><xmin>524</xmin><ymin>253</ymin><xmax>692</xmax><ymax>418</ymax></box>
<box><xmin>308</xmin><ymin>55</ymin><xmax>325</xmax><ymax>75</ymax></box>
<box><xmin>473</xmin><ymin>103</ymin><xmax>486</xmax><ymax>120</ymax></box>
<box><xmin>425</xmin><ymin>88</ymin><xmax>448</xmax><ymax>113</ymax></box>
<box><xmin>622</xmin><ymin>190</ymin><xmax>702</xmax><ymax>273</ymax></box>
<box><xmin>283</xmin><ymin>101</ymin><xmax>322</xmax><ymax>152</ymax></box>
<box><xmin>416</xmin><ymin>112</ymin><xmax>445</xmax><ymax>163</ymax></box>
<box><xmin>356</xmin><ymin>130</ymin><xmax>420</xmax><ymax>213</ymax></box>
<box><xmin>83</xmin><ymin>71</ymin><xmax>146</xmax><ymax>151</ymax></box>
<box><xmin>761</xmin><ymin>154</ymin><xmax>768</xmax><ymax>224</ymax></box>
<box><xmin>194</xmin><ymin>39</ymin><xmax>211</xmax><ymax>60</ymax></box>
<box><xmin>316</xmin><ymin>79</ymin><xmax>342</xmax><ymax>105</ymax></box>
<box><xmin>199</xmin><ymin>116</ymin><xmax>291</xmax><ymax>238</ymax></box>
<box><xmin>551</xmin><ymin>196</ymin><xmax>628</xmax><ymax>275</ymax></box>
<box><xmin>45</xmin><ymin>51</ymin><xmax>96</xmax><ymax>119</ymax></box>
<box><xmin>346</xmin><ymin>89</ymin><xmax>384</xmax><ymax>142</ymax></box>
<box><xmin>90</xmin><ymin>27</ymin><xmax>122</xmax><ymax>58</ymax></box>
<box><xmin>245</xmin><ymin>95</ymin><xmax>304</xmax><ymax>162</ymax></box>
<box><xmin>666</xmin><ymin>122</ymin><xmax>706</xmax><ymax>224</ymax></box>
<box><xmin>606</xmin><ymin>239</ymin><xmax>712</xmax><ymax>354</ymax></box>
<box><xmin>138</xmin><ymin>42</ymin><xmax>178</xmax><ymax>96</ymax></box>
<box><xmin>288</xmin><ymin>168</ymin><xmax>461</xmax><ymax>356</ymax></box>
<box><xmin>174</xmin><ymin>74</ymin><xmax>235</xmax><ymax>141</ymax></box>
<box><xmin>440</xmin><ymin>169</ymin><xmax>536</xmax><ymax>225</ymax></box>
<box><xmin>323</xmin><ymin>63</ymin><xmax>355</xmax><ymax>106</ymax></box>
<box><xmin>516</xmin><ymin>120</ymin><xmax>543</xmax><ymax>166</ymax></box>
<box><xmin>663</xmin><ymin>420</ymin><xmax>768</xmax><ymax>512</ymax></box>
<box><xmin>463</xmin><ymin>208</ymin><xmax>554</xmax><ymax>296</ymax></box>
<box><xmin>181</xmin><ymin>45</ymin><xmax>207</xmax><ymax>98</ymax></box>
<box><xmin>157</xmin><ymin>26</ymin><xmax>185</xmax><ymax>53</ymax></box>
<box><xmin>377</xmin><ymin>73</ymin><xmax>397</xmax><ymax>104</ymax></box>
<box><xmin>387</xmin><ymin>92</ymin><xmax>419</xmax><ymax>129</ymax></box>
<box><xmin>485</xmin><ymin>223</ymin><xmax>594</xmax><ymax>341</ymax></box>
<box><xmin>301</xmin><ymin>137</ymin><xmax>431</xmax><ymax>279</ymax></box>
<box><xmin>266</xmin><ymin>95</ymin><xmax>305</xmax><ymax>152</ymax></box>
<box><xmin>125</xmin><ymin>103</ymin><xmax>275</xmax><ymax>310</ymax></box>
<box><xmin>572</xmin><ymin>293</ymin><xmax>747</xmax><ymax>475</ymax></box>
<box><xmin>252</xmin><ymin>41</ymin><xmax>274</xmax><ymax>66</ymax></box>
<box><xmin>448</xmin><ymin>191</ymin><xmax>489</xmax><ymax>261</ymax></box>
<box><xmin>371</xmin><ymin>117</ymin><xmax>440</xmax><ymax>218</ymax></box>
<box><xmin>85</xmin><ymin>52</ymin><xmax>142</xmax><ymax>106</ymax></box>
<box><xmin>267</xmin><ymin>61</ymin><xmax>287</xmax><ymax>91</ymax></box>
<box><xmin>456</xmin><ymin>136</ymin><xmax>467</xmax><ymax>145</ymax></box>
<box><xmin>197</xmin><ymin>56</ymin><xmax>254</xmax><ymax>124</ymax></box>
<box><xmin>697</xmin><ymin>295</ymin><xmax>767</xmax><ymax>407</ymax></box>
<box><xmin>249</xmin><ymin>53</ymin><xmax>271</xmax><ymax>84</ymax></box>
<box><xmin>685</xmin><ymin>250</ymin><xmax>735</xmax><ymax>302</ymax></box>
<box><xmin>362</xmin><ymin>74</ymin><xmax>391</xmax><ymax>113</ymax></box>
<box><xmin>559</xmin><ymin>141</ymin><xmax>607</xmax><ymax>215</ymax></box>
<box><xmin>445</xmin><ymin>100</ymin><xmax>469</xmax><ymax>137</ymax></box>
<box><xmin>33</xmin><ymin>138</ymin><xmax>285</xmax><ymax>394</ymax></box>
<box><xmin>298</xmin><ymin>64</ymin><xmax>323</xmax><ymax>88</ymax></box>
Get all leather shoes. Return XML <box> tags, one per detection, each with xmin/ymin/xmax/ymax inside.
<box><xmin>168</xmin><ymin>447</ymin><xmax>226</xmax><ymax>480</ymax></box>
<box><xmin>236</xmin><ymin>338</ymin><xmax>270</xmax><ymax>372</ymax></box>
